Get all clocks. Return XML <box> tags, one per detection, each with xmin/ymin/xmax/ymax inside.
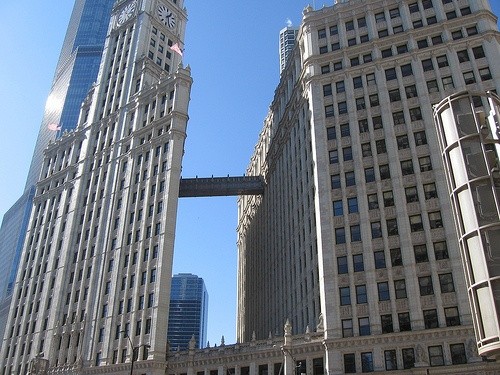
<box><xmin>117</xmin><ymin>2</ymin><xmax>136</xmax><ymax>26</ymax></box>
<box><xmin>156</xmin><ymin>4</ymin><xmax>178</xmax><ymax>32</ymax></box>
